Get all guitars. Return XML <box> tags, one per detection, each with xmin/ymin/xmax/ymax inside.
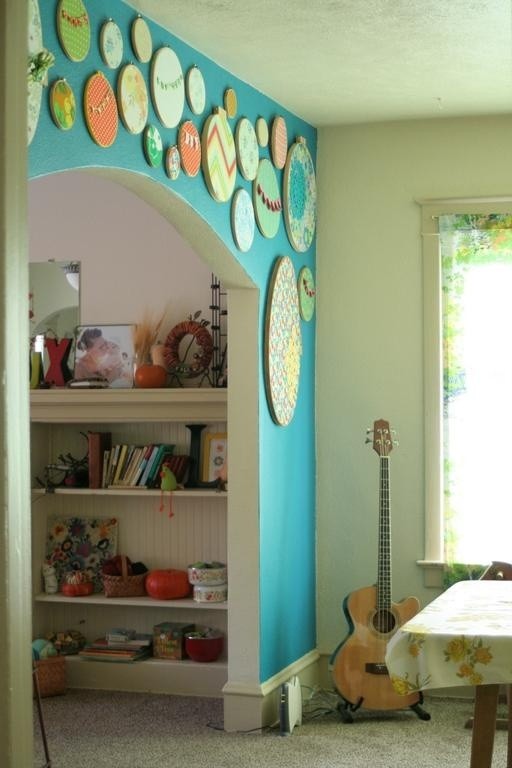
<box><xmin>330</xmin><ymin>419</ymin><xmax>420</xmax><ymax>710</ymax></box>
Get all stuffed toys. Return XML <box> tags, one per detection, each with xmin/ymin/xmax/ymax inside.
<box><xmin>156</xmin><ymin>463</ymin><xmax>178</xmax><ymax>520</ymax></box>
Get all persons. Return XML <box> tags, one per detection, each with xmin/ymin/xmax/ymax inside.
<box><xmin>76</xmin><ymin>326</ymin><xmax>131</xmax><ymax>381</ymax></box>
<box><xmin>99</xmin><ymin>335</ymin><xmax>128</xmax><ymax>375</ymax></box>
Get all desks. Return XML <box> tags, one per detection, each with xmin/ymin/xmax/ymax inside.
<box><xmin>394</xmin><ymin>579</ymin><xmax>512</xmax><ymax>767</ymax></box>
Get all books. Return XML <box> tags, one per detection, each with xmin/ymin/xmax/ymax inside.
<box><xmin>86</xmin><ymin>429</ymin><xmax>190</xmax><ymax>490</ymax></box>
<box><xmin>77</xmin><ymin>626</ymin><xmax>153</xmax><ymax>663</ymax></box>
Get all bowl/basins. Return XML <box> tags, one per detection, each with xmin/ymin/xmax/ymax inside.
<box><xmin>185</xmin><ymin>631</ymin><xmax>225</xmax><ymax>663</ymax></box>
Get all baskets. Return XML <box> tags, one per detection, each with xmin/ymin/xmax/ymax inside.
<box><xmin>32</xmin><ymin>657</ymin><xmax>65</xmax><ymax>698</ymax></box>
<box><xmin>99</xmin><ymin>551</ymin><xmax>149</xmax><ymax>598</ymax></box>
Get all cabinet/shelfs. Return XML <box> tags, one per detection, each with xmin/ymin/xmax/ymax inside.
<box><xmin>28</xmin><ymin>388</ymin><xmax>230</xmax><ymax>697</ymax></box>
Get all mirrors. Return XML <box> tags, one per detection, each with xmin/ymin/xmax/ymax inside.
<box><xmin>28</xmin><ymin>259</ymin><xmax>81</xmax><ymax>386</ymax></box>
